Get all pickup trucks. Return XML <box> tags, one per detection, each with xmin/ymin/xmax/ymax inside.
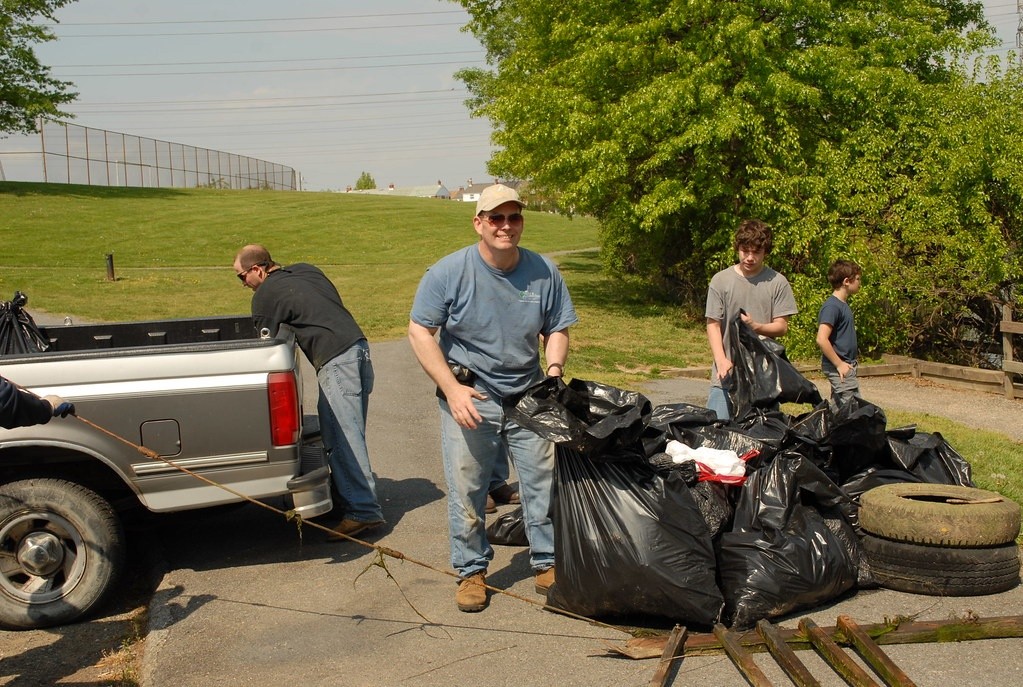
<box><xmin>0</xmin><ymin>315</ymin><xmax>334</xmax><ymax>631</ymax></box>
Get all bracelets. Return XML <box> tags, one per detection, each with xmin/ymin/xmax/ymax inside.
<box><xmin>546</xmin><ymin>363</ymin><xmax>565</xmax><ymax>377</ymax></box>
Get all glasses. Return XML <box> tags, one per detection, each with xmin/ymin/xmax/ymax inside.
<box><xmin>237</xmin><ymin>261</ymin><xmax>267</xmax><ymax>281</ymax></box>
<box><xmin>478</xmin><ymin>213</ymin><xmax>523</xmax><ymax>228</ymax></box>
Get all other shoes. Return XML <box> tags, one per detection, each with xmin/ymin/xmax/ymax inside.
<box><xmin>328</xmin><ymin>517</ymin><xmax>386</xmax><ymax>539</ymax></box>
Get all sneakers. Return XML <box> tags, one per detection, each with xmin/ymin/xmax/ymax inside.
<box><xmin>534</xmin><ymin>565</ymin><xmax>556</xmax><ymax>595</ymax></box>
<box><xmin>483</xmin><ymin>493</ymin><xmax>496</xmax><ymax>513</ymax></box>
<box><xmin>456</xmin><ymin>570</ymin><xmax>488</xmax><ymax>611</ymax></box>
<box><xmin>489</xmin><ymin>482</ymin><xmax>521</xmax><ymax>504</ymax></box>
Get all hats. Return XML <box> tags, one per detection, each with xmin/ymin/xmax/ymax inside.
<box><xmin>476</xmin><ymin>184</ymin><xmax>527</xmax><ymax>216</ymax></box>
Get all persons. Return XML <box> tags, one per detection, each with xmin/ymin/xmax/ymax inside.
<box><xmin>484</xmin><ymin>444</ymin><xmax>522</xmax><ymax>514</ymax></box>
<box><xmin>408</xmin><ymin>184</ymin><xmax>579</xmax><ymax>612</ymax></box>
<box><xmin>705</xmin><ymin>220</ymin><xmax>798</xmax><ymax>419</ymax></box>
<box><xmin>815</xmin><ymin>259</ymin><xmax>862</xmax><ymax>414</ymax></box>
<box><xmin>234</xmin><ymin>245</ymin><xmax>388</xmax><ymax>539</ymax></box>
